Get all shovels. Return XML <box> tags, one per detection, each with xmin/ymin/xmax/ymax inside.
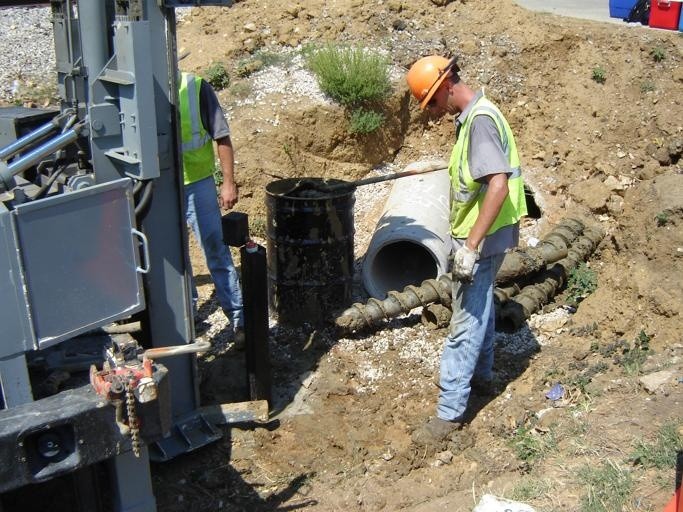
<box><xmin>283</xmin><ymin>165</ymin><xmax>450</xmax><ymax>197</ymax></box>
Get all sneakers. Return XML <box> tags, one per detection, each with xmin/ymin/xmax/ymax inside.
<box><xmin>433</xmin><ymin>379</ymin><xmax>491</xmax><ymax>396</ymax></box>
<box><xmin>411</xmin><ymin>416</ymin><xmax>463</xmax><ymax>446</ymax></box>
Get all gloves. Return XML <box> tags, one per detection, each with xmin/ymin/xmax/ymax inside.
<box><xmin>448</xmin><ymin>242</ymin><xmax>477</xmax><ymax>284</ymax></box>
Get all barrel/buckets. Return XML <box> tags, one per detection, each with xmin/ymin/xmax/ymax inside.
<box><xmin>264</xmin><ymin>178</ymin><xmax>355</xmax><ymax>319</ymax></box>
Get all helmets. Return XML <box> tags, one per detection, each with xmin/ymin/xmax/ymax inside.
<box><xmin>406</xmin><ymin>55</ymin><xmax>459</xmax><ymax>112</ymax></box>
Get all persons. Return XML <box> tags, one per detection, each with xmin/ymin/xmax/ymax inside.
<box><xmin>408</xmin><ymin>54</ymin><xmax>529</xmax><ymax>439</ymax></box>
<box><xmin>175</xmin><ymin>68</ymin><xmax>246</xmax><ymax>350</ymax></box>
